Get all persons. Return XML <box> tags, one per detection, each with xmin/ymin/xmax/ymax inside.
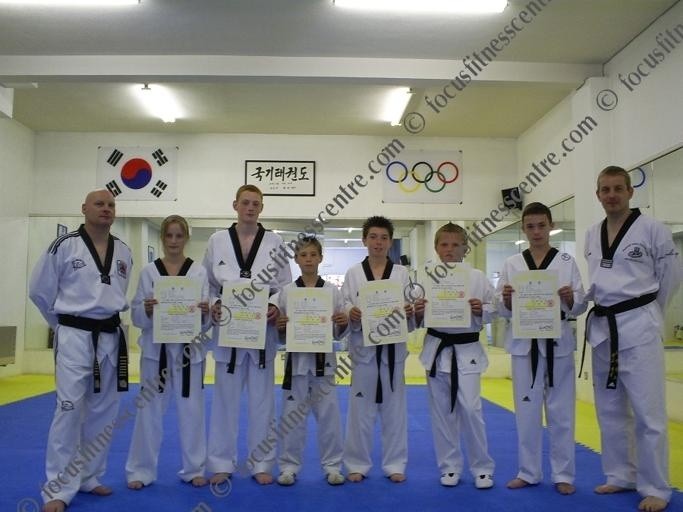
<box><xmin>413</xmin><ymin>224</ymin><xmax>498</xmax><ymax>489</ymax></box>
<box><xmin>579</xmin><ymin>166</ymin><xmax>681</xmax><ymax>512</ymax></box>
<box><xmin>275</xmin><ymin>236</ymin><xmax>350</xmax><ymax>485</ymax></box>
<box><xmin>124</xmin><ymin>215</ymin><xmax>211</xmax><ymax>489</ymax></box>
<box><xmin>28</xmin><ymin>190</ymin><xmax>134</xmax><ymax>512</ymax></box>
<box><xmin>495</xmin><ymin>202</ymin><xmax>589</xmax><ymax>495</ymax></box>
<box><xmin>341</xmin><ymin>217</ymin><xmax>414</xmax><ymax>482</ymax></box>
<box><xmin>201</xmin><ymin>185</ymin><xmax>292</xmax><ymax>485</ymax></box>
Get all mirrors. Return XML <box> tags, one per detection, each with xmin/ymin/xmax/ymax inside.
<box><xmin>24</xmin><ymin>216</ymin><xmax>519</xmax><ymax>352</ymax></box>
<box><xmin>627</xmin><ymin>146</ymin><xmax>683</xmax><ymax>424</ymax></box>
<box><xmin>518</xmin><ymin>194</ymin><xmax>577</xmax><ymax>353</ymax></box>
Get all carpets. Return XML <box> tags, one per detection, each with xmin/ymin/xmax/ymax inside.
<box><xmin>0</xmin><ymin>383</ymin><xmax>682</xmax><ymax>512</ymax></box>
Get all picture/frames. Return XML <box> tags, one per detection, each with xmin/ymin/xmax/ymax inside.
<box><xmin>58</xmin><ymin>224</ymin><xmax>67</xmax><ymax>237</ymax></box>
<box><xmin>148</xmin><ymin>246</ymin><xmax>154</xmax><ymax>262</ymax></box>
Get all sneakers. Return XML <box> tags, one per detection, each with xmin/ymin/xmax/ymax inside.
<box><xmin>277</xmin><ymin>472</ymin><xmax>296</xmax><ymax>486</ymax></box>
<box><xmin>474</xmin><ymin>475</ymin><xmax>492</xmax><ymax>490</ymax></box>
<box><xmin>325</xmin><ymin>471</ymin><xmax>344</xmax><ymax>485</ymax></box>
<box><xmin>439</xmin><ymin>473</ymin><xmax>461</xmax><ymax>487</ymax></box>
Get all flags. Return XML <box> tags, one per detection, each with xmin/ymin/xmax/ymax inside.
<box><xmin>380</xmin><ymin>149</ymin><xmax>465</xmax><ymax>205</ymax></box>
<box><xmin>94</xmin><ymin>146</ymin><xmax>180</xmax><ymax>203</ymax></box>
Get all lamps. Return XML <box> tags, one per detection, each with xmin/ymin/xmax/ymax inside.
<box><xmin>141</xmin><ymin>83</ymin><xmax>176</xmax><ymax>123</ymax></box>
<box><xmin>390</xmin><ymin>87</ymin><xmax>416</xmax><ymax>127</ymax></box>
<box><xmin>334</xmin><ymin>0</ymin><xmax>511</xmax><ymax>12</ymax></box>
